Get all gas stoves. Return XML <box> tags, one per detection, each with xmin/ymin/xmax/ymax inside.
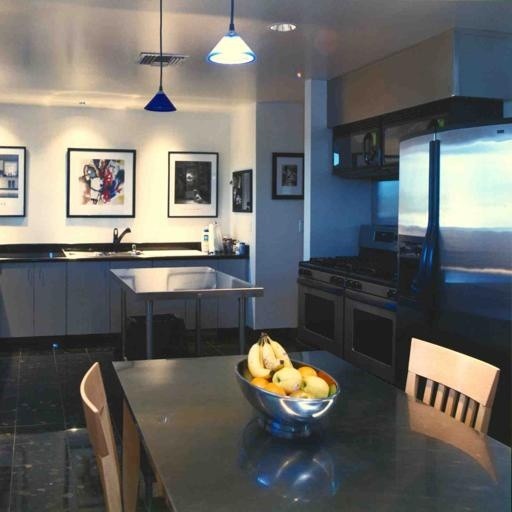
<box><xmin>299</xmin><ymin>223</ymin><xmax>398</xmax><ymax>301</ymax></box>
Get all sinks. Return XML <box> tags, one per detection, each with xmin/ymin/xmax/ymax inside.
<box><xmin>105</xmin><ymin>251</ymin><xmax>143</xmax><ymax>257</ymax></box>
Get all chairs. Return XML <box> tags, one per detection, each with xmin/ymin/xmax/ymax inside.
<box><xmin>404</xmin><ymin>337</ymin><xmax>501</xmax><ymax>434</ymax></box>
<box><xmin>79</xmin><ymin>362</ymin><xmax>123</xmax><ymax>512</ymax></box>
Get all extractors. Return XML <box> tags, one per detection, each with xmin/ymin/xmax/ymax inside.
<box><xmin>319</xmin><ymin>25</ymin><xmax>511</xmax><ymax>183</ymax></box>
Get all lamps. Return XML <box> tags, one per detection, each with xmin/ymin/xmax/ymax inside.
<box><xmin>207</xmin><ymin>0</ymin><xmax>257</xmax><ymax>65</ymax></box>
<box><xmin>144</xmin><ymin>0</ymin><xmax>177</xmax><ymax>111</ymax></box>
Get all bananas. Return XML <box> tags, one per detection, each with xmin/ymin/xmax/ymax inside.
<box><xmin>248</xmin><ymin>333</ymin><xmax>294</xmax><ymax>379</ymax></box>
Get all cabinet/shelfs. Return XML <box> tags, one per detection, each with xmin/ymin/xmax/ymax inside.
<box><xmin>153</xmin><ymin>258</ymin><xmax>249</xmax><ymax>330</ymax></box>
<box><xmin>66</xmin><ymin>260</ymin><xmax>153</xmax><ymax>336</ymax></box>
<box><xmin>1</xmin><ymin>261</ymin><xmax>66</xmax><ymax>338</ymax></box>
<box><xmin>332</xmin><ymin>102</ymin><xmax>503</xmax><ymax>179</ymax></box>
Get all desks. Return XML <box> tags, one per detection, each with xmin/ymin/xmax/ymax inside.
<box><xmin>108</xmin><ymin>266</ymin><xmax>265</xmax><ymax>361</ymax></box>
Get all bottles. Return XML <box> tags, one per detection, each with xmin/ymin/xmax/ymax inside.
<box><xmin>222</xmin><ymin>237</ymin><xmax>246</xmax><ymax>256</ymax></box>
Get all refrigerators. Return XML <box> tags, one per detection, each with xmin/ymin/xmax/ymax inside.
<box><xmin>395</xmin><ymin>124</ymin><xmax>512</xmax><ymax>452</ymax></box>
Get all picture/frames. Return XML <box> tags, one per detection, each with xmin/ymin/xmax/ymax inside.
<box><xmin>0</xmin><ymin>146</ymin><xmax>26</xmax><ymax>217</ymax></box>
<box><xmin>168</xmin><ymin>151</ymin><xmax>218</xmax><ymax>218</ymax></box>
<box><xmin>232</xmin><ymin>169</ymin><xmax>252</xmax><ymax>212</ymax></box>
<box><xmin>271</xmin><ymin>152</ymin><xmax>304</xmax><ymax>199</ymax></box>
<box><xmin>67</xmin><ymin>148</ymin><xmax>136</xmax><ymax>217</ymax></box>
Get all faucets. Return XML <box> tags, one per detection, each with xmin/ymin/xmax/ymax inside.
<box><xmin>112</xmin><ymin>227</ymin><xmax>131</xmax><ymax>251</ymax></box>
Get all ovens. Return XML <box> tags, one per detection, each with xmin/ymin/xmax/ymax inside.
<box><xmin>296</xmin><ymin>273</ymin><xmax>398</xmax><ymax>400</ymax></box>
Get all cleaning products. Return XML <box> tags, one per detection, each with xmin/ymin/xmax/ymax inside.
<box><xmin>202</xmin><ymin>225</ymin><xmax>210</xmax><ymax>252</ymax></box>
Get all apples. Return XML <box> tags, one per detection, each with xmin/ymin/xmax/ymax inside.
<box><xmin>273</xmin><ymin>367</ymin><xmax>304</xmax><ymax>393</ymax></box>
<box><xmin>301</xmin><ymin>375</ymin><xmax>329</xmax><ymax>399</ymax></box>
<box><xmin>290</xmin><ymin>392</ymin><xmax>316</xmax><ymax>399</ymax></box>
